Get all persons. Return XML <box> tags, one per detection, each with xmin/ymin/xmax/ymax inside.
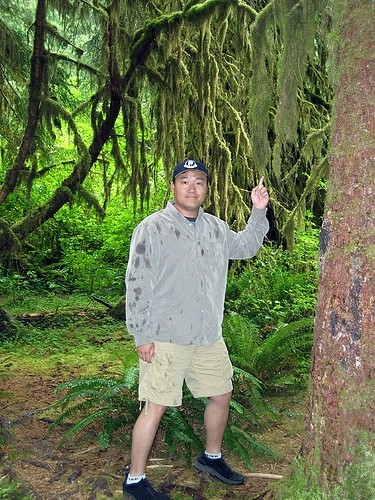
<box><xmin>124</xmin><ymin>157</ymin><xmax>270</xmax><ymax>500</ymax></box>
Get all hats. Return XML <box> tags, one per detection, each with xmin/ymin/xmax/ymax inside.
<box><xmin>172</xmin><ymin>158</ymin><xmax>208</xmax><ymax>179</ymax></box>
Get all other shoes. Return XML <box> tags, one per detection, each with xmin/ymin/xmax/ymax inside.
<box><xmin>122</xmin><ymin>476</ymin><xmax>169</xmax><ymax>500</ymax></box>
<box><xmin>193</xmin><ymin>451</ymin><xmax>245</xmax><ymax>485</ymax></box>
